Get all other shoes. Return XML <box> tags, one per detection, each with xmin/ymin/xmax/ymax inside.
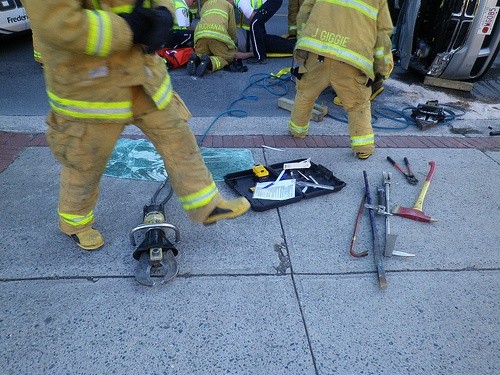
<box><xmin>196</xmin><ymin>54</ymin><xmax>211</xmax><ymax>78</ymax></box>
<box><xmin>333</xmin><ymin>96</ymin><xmax>343</xmax><ymax>106</ymax></box>
<box><xmin>247</xmin><ymin>57</ymin><xmax>268</xmax><ymax>64</ymax></box>
<box><xmin>186</xmin><ymin>52</ymin><xmax>198</xmax><ymax>76</ymax></box>
<box><xmin>370</xmin><ymin>83</ymin><xmax>385</xmax><ymax>101</ymax></box>
<box><xmin>357</xmin><ymin>152</ymin><xmax>371</xmax><ymax>159</ymax></box>
<box><xmin>65</xmin><ymin>226</ymin><xmax>105</xmax><ymax>250</ymax></box>
<box><xmin>202</xmin><ymin>197</ymin><xmax>251</xmax><ymax>226</ymax></box>
<box><xmin>293</xmin><ymin>131</ymin><xmax>305</xmax><ymax>139</ymax></box>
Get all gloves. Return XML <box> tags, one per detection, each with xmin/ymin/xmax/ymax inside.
<box><xmin>118</xmin><ymin>0</ymin><xmax>174</xmax><ymax>55</ymax></box>
<box><xmin>374</xmin><ymin>73</ymin><xmax>383</xmax><ymax>82</ymax></box>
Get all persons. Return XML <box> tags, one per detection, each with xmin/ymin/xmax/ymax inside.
<box><xmin>21</xmin><ymin>0</ymin><xmax>251</xmax><ymax>250</ymax></box>
<box><xmin>171</xmin><ymin>0</ymin><xmax>394</xmax><ymax>159</ymax></box>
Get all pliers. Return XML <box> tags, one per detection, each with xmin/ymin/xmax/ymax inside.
<box><xmin>129</xmin><ymin>203</ymin><xmax>180</xmax><ymax>286</ymax></box>
<box><xmin>387</xmin><ymin>156</ymin><xmax>419</xmax><ymax>185</ymax></box>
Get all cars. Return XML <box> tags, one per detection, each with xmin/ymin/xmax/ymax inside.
<box><xmin>394</xmin><ymin>0</ymin><xmax>500</xmax><ymax>81</ymax></box>
<box><xmin>0</xmin><ymin>0</ymin><xmax>32</xmax><ymax>40</ymax></box>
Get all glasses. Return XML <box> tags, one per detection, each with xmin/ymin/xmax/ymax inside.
<box><xmin>193</xmin><ymin>0</ymin><xmax>195</xmax><ymax>2</ymax></box>
